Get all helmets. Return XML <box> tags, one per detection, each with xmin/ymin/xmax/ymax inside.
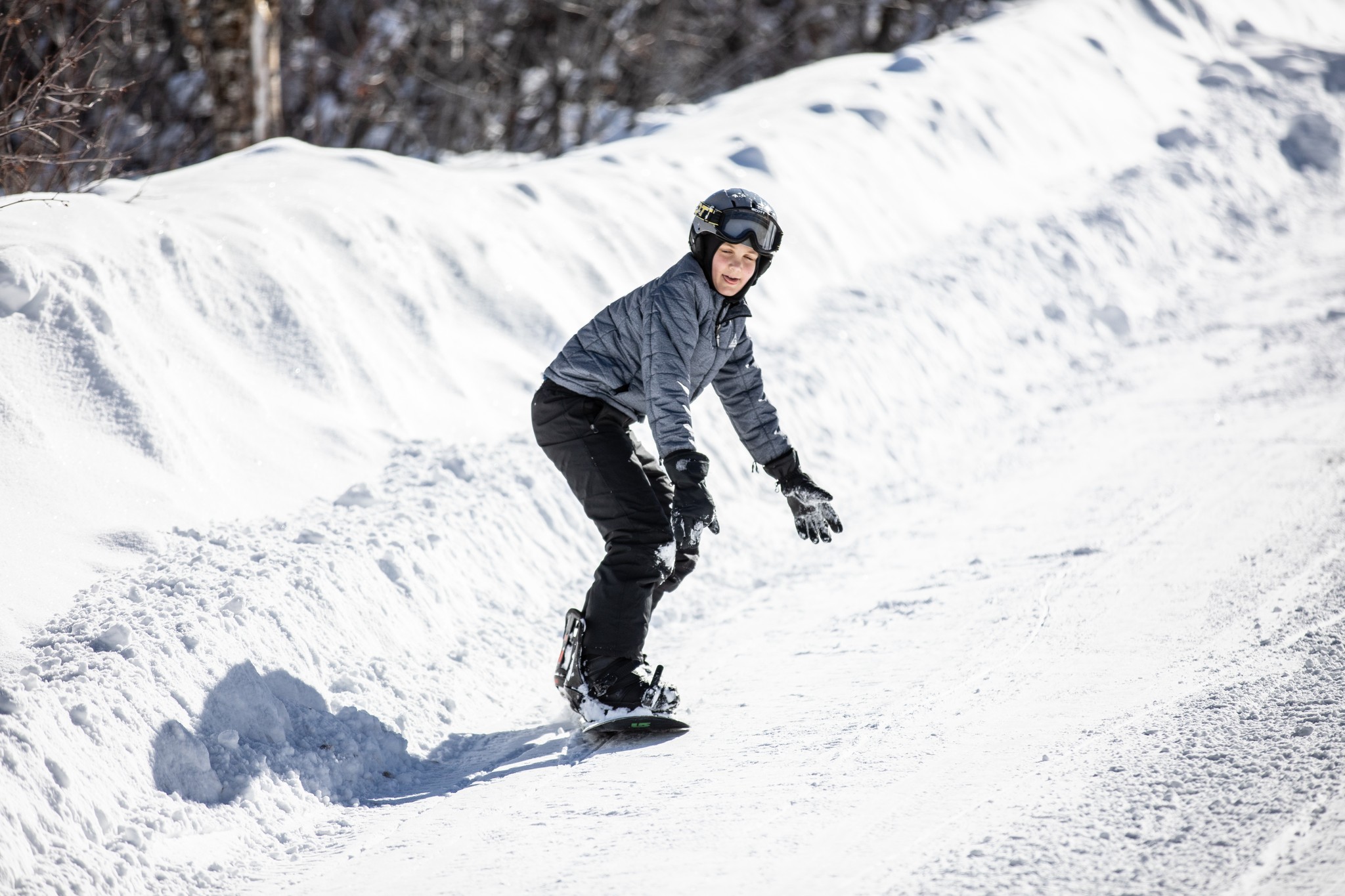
<box><xmin>689</xmin><ymin>188</ymin><xmax>778</xmax><ymax>286</ymax></box>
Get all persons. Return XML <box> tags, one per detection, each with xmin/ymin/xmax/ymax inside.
<box><xmin>530</xmin><ymin>188</ymin><xmax>843</xmax><ymax>713</ymax></box>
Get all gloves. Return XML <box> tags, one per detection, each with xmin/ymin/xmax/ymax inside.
<box><xmin>763</xmin><ymin>448</ymin><xmax>843</xmax><ymax>544</ymax></box>
<box><xmin>663</xmin><ymin>450</ymin><xmax>720</xmax><ymax>555</ymax></box>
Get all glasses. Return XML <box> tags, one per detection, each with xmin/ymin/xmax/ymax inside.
<box><xmin>694</xmin><ymin>201</ymin><xmax>783</xmax><ymax>254</ymax></box>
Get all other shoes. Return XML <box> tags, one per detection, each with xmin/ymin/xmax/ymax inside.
<box><xmin>582</xmin><ymin>654</ymin><xmax>680</xmax><ymax>715</ymax></box>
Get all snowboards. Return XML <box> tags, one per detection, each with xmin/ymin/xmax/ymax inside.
<box><xmin>577</xmin><ymin>715</ymin><xmax>692</xmax><ymax>733</ymax></box>
